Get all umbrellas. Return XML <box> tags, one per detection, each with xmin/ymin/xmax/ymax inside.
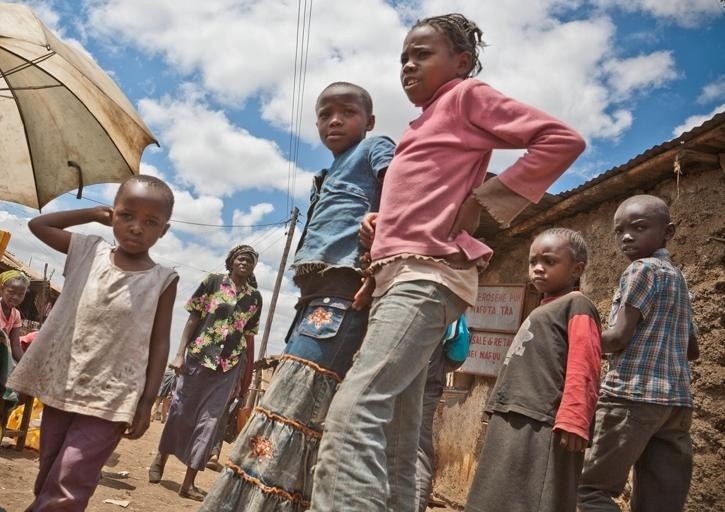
<box><xmin>0</xmin><ymin>0</ymin><xmax>161</xmax><ymax>214</ymax></box>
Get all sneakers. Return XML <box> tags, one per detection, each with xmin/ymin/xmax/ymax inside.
<box><xmin>148</xmin><ymin>453</ymin><xmax>170</xmax><ymax>484</ymax></box>
<box><xmin>206</xmin><ymin>459</ymin><xmax>219</xmax><ymax>469</ymax></box>
<box><xmin>178</xmin><ymin>484</ymin><xmax>206</xmax><ymax>502</ymax></box>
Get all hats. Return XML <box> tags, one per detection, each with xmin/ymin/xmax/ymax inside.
<box><xmin>225</xmin><ymin>244</ymin><xmax>260</xmax><ymax>271</ymax></box>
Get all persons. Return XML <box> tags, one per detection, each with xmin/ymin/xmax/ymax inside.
<box><xmin>0</xmin><ymin>269</ymin><xmax>28</xmax><ymax>432</ymax></box>
<box><xmin>149</xmin><ymin>245</ymin><xmax>262</xmax><ymax>502</ymax></box>
<box><xmin>195</xmin><ymin>81</ymin><xmax>397</xmax><ymax>512</ymax></box>
<box><xmin>576</xmin><ymin>195</ymin><xmax>700</xmax><ymax>511</ymax></box>
<box><xmin>151</xmin><ymin>370</ymin><xmax>177</xmax><ymax>424</ymax></box>
<box><xmin>308</xmin><ymin>13</ymin><xmax>584</xmax><ymax>511</ymax></box>
<box><xmin>207</xmin><ymin>384</ymin><xmax>242</xmax><ymax>468</ymax></box>
<box><xmin>1</xmin><ymin>175</ymin><xmax>179</xmax><ymax>512</ymax></box>
<box><xmin>463</xmin><ymin>228</ymin><xmax>602</xmax><ymax>511</ymax></box>
